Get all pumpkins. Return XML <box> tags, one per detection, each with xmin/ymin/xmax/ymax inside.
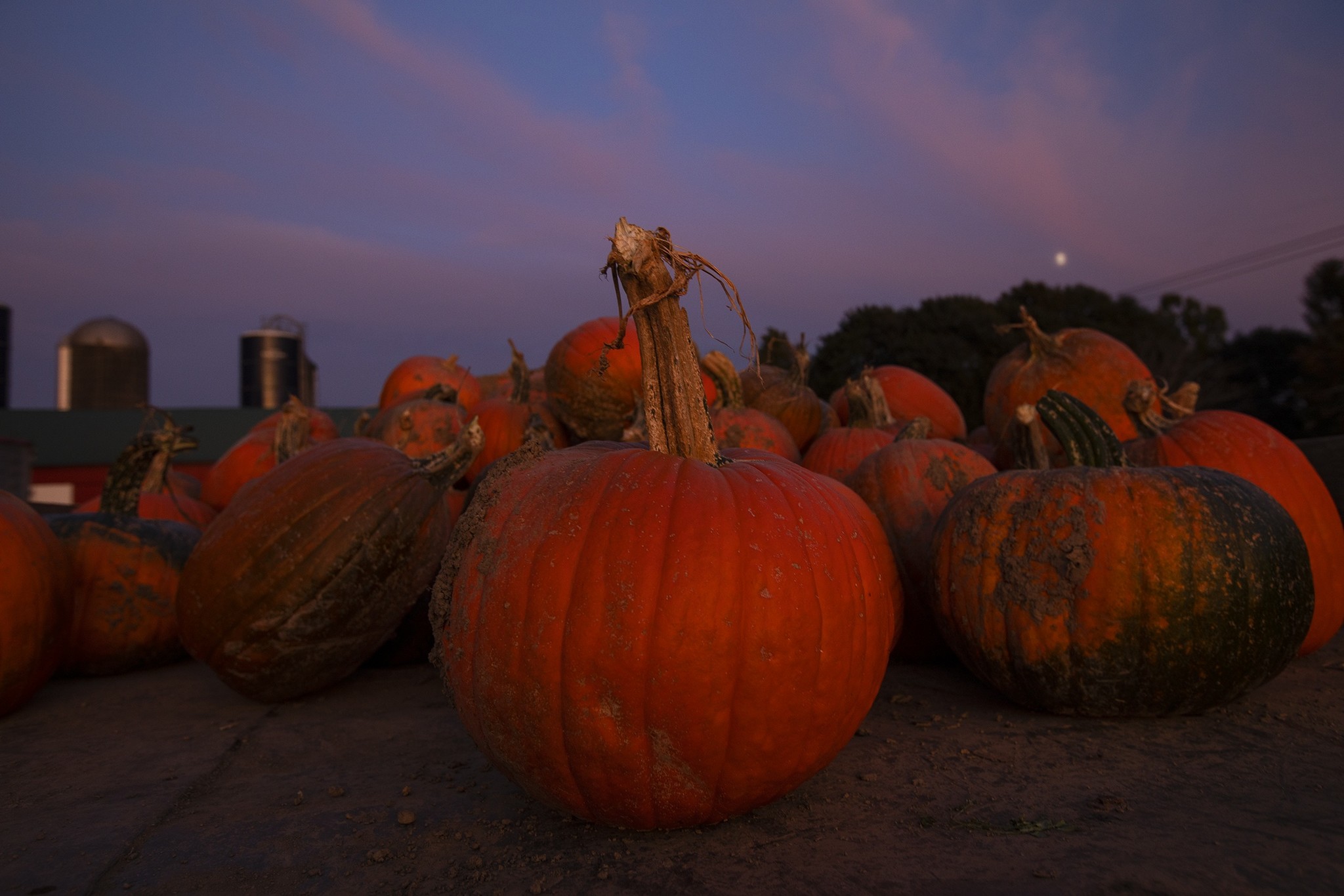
<box><xmin>0</xmin><ymin>213</ymin><xmax>1344</xmax><ymax>834</ymax></box>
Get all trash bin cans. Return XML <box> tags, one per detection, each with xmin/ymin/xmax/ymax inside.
<box><xmin>58</xmin><ymin>319</ymin><xmax>148</xmax><ymax>411</ymax></box>
<box><xmin>240</xmin><ymin>331</ymin><xmax>305</xmax><ymax>411</ymax></box>
